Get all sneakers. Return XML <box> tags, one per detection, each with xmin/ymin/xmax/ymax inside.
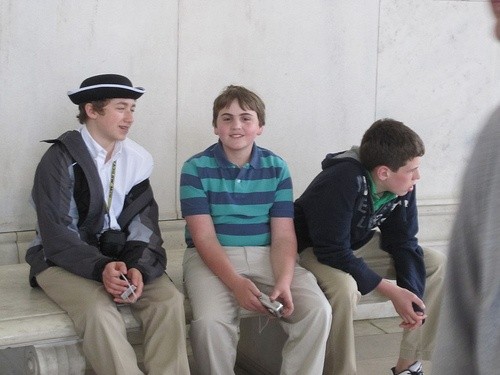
<box><xmin>390</xmin><ymin>360</ymin><xmax>423</xmax><ymax>375</ymax></box>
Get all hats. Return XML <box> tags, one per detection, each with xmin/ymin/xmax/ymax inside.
<box><xmin>65</xmin><ymin>74</ymin><xmax>145</xmax><ymax>105</ymax></box>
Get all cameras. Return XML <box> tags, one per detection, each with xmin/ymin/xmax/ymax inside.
<box><xmin>119</xmin><ymin>276</ymin><xmax>137</xmax><ymax>300</ymax></box>
<box><xmin>257</xmin><ymin>292</ymin><xmax>284</xmax><ymax>319</ymax></box>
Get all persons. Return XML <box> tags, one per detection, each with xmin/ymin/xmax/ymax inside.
<box><xmin>293</xmin><ymin>118</ymin><xmax>429</xmax><ymax>375</ymax></box>
<box><xmin>25</xmin><ymin>74</ymin><xmax>191</xmax><ymax>375</ymax></box>
<box><xmin>430</xmin><ymin>0</ymin><xmax>499</xmax><ymax>375</ymax></box>
<box><xmin>180</xmin><ymin>86</ymin><xmax>333</xmax><ymax>375</ymax></box>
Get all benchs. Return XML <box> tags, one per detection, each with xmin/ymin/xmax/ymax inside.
<box><xmin>0</xmin><ymin>250</ymin><xmax>397</xmax><ymax>375</ymax></box>
